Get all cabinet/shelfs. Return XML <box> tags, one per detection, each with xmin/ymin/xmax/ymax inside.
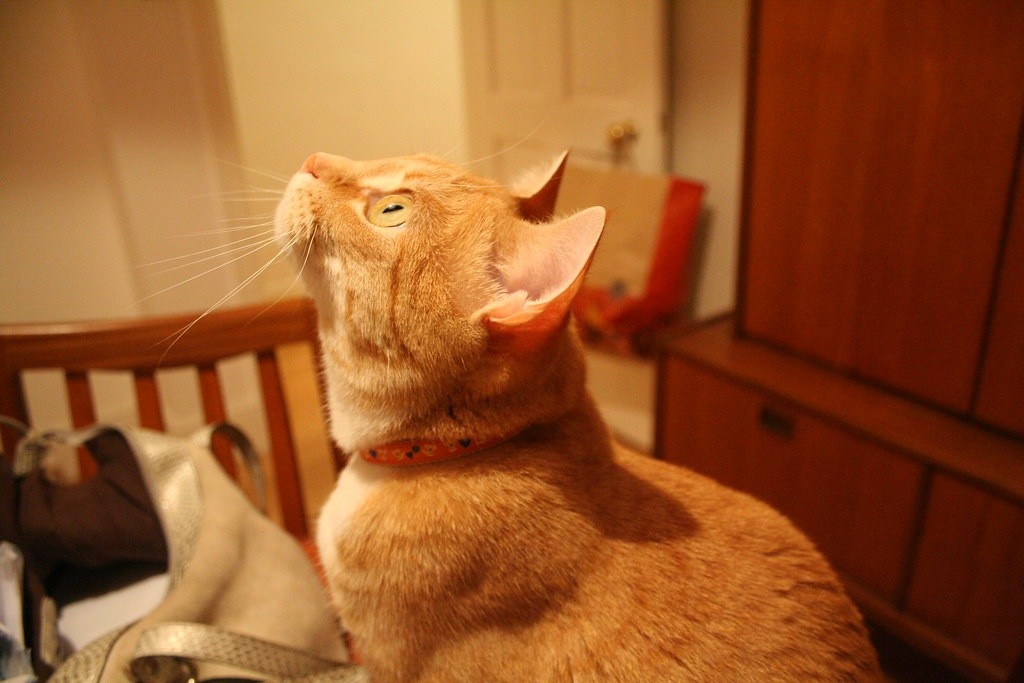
<box><xmin>737</xmin><ymin>0</ymin><xmax>1024</xmax><ymax>445</ymax></box>
<box><xmin>649</xmin><ymin>315</ymin><xmax>1024</xmax><ymax>683</ymax></box>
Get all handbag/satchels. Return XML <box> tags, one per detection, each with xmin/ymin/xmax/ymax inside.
<box><xmin>0</xmin><ymin>413</ymin><xmax>359</xmax><ymax>683</ymax></box>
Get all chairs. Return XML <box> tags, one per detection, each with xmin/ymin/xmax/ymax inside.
<box><xmin>0</xmin><ymin>299</ymin><xmax>347</xmax><ymax>576</ymax></box>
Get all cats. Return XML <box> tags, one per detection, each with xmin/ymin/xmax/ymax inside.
<box><xmin>274</xmin><ymin>148</ymin><xmax>880</xmax><ymax>683</ymax></box>
